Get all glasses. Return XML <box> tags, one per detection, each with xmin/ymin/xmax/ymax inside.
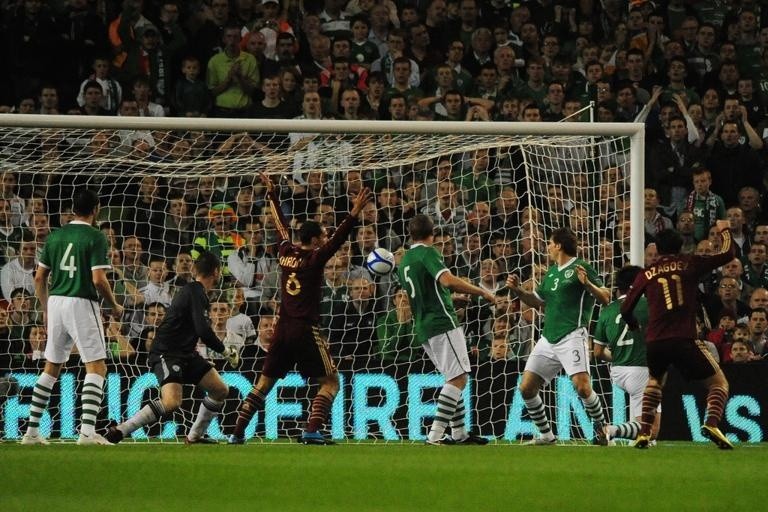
<box><xmin>326</xmin><ymin>266</ymin><xmax>342</xmax><ymax>269</ymax></box>
<box><xmin>542</xmin><ymin>41</ymin><xmax>559</xmax><ymax>46</ymax></box>
<box><xmin>718</xmin><ymin>284</ymin><xmax>736</xmax><ymax>288</ymax></box>
<box><xmin>316</xmin><ymin>212</ymin><xmax>334</xmax><ymax>216</ymax></box>
<box><xmin>597</xmin><ymin>87</ymin><xmax>609</xmax><ymax>94</ymax></box>
<box><xmin>679</xmin><ymin>218</ymin><xmax>694</xmax><ymax>223</ymax></box>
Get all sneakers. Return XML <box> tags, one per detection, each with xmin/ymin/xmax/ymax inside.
<box><xmin>629</xmin><ymin>441</ymin><xmax>657</xmax><ymax>446</ymax></box>
<box><xmin>186</xmin><ymin>437</ymin><xmax>219</xmax><ymax>445</ymax></box>
<box><xmin>228</xmin><ymin>434</ymin><xmax>245</xmax><ymax>444</ymax></box>
<box><xmin>424</xmin><ymin>435</ymin><xmax>477</xmax><ymax>447</ymax></box>
<box><xmin>451</xmin><ymin>432</ymin><xmax>489</xmax><ymax>445</ymax></box>
<box><xmin>634</xmin><ymin>432</ymin><xmax>651</xmax><ymax>448</ymax></box>
<box><xmin>21</xmin><ymin>434</ymin><xmax>50</xmax><ymax>445</ymax></box>
<box><xmin>700</xmin><ymin>424</ymin><xmax>734</xmax><ymax>450</ymax></box>
<box><xmin>77</xmin><ymin>434</ymin><xmax>115</xmax><ymax>446</ymax></box>
<box><xmin>301</xmin><ymin>432</ymin><xmax>340</xmax><ymax>446</ymax></box>
<box><xmin>104</xmin><ymin>426</ymin><xmax>124</xmax><ymax>444</ymax></box>
<box><xmin>523</xmin><ymin>437</ymin><xmax>555</xmax><ymax>446</ymax></box>
<box><xmin>594</xmin><ymin>421</ymin><xmax>609</xmax><ymax>447</ymax></box>
<box><xmin>609</xmin><ymin>438</ymin><xmax>616</xmax><ymax>446</ymax></box>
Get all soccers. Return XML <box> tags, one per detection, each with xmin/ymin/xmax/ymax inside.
<box><xmin>367</xmin><ymin>248</ymin><xmax>395</xmax><ymax>275</ymax></box>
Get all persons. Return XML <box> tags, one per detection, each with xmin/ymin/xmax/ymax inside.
<box><xmin>298</xmin><ymin>15</ymin><xmax>322</xmax><ymax>62</ymax></box>
<box><xmin>507</xmin><ymin>228</ymin><xmax>614</xmax><ymax>446</ymax></box>
<box><xmin>521</xmin><ymin>103</ymin><xmax>543</xmax><ymax>122</ymax></box>
<box><xmin>453</xmin><ymin>149</ymin><xmax>498</xmax><ymax>207</ymax></box>
<box><xmin>541</xmin><ymin>80</ymin><xmax>567</xmax><ymax>173</ymax></box>
<box><xmin>696</xmin><ymin>318</ymin><xmax>720</xmax><ymax>363</ymax></box>
<box><xmin>511</xmin><ymin>279</ymin><xmax>547</xmax><ymax>358</ymax></box>
<box><xmin>423</xmin><ymin>156</ymin><xmax>469</xmax><ymax>205</ymax></box>
<box><xmin>597</xmin><ymin>239</ymin><xmax>621</xmax><ymax>315</ymax></box>
<box><xmin>489</xmin><ymin>335</ymin><xmax>511</xmax><ymax>360</ymax></box>
<box><xmin>369</xmin><ymin>1</ymin><xmax>401</xmax><ymax>41</ymax></box>
<box><xmin>714</xmin><ymin>59</ymin><xmax>742</xmax><ymax>99</ymax></box>
<box><xmin>169</xmin><ymin>55</ymin><xmax>215</xmax><ymax>117</ymax></box>
<box><xmin>634</xmin><ymin>86</ymin><xmax>699</xmax><ymax>147</ymax></box>
<box><xmin>605</xmin><ymin>49</ymin><xmax>628</xmax><ymax>81</ymax></box>
<box><xmin>708</xmin><ymin>227</ymin><xmax>734</xmax><ymax>246</ymax></box>
<box><xmin>460</xmin><ymin>233</ymin><xmax>489</xmax><ymax>277</ymax></box>
<box><xmin>403</xmin><ymin>173</ymin><xmax>425</xmax><ymax>208</ymax></box>
<box><xmin>522</xmin><ymin>208</ymin><xmax>544</xmax><ymax>231</ymax></box>
<box><xmin>109</xmin><ymin>0</ymin><xmax>154</xmax><ymax>101</ymax></box>
<box><xmin>490</xmin><ymin>236</ymin><xmax>521</xmax><ymax>281</ymax></box>
<box><xmin>742</xmin><ymin>23</ymin><xmax>767</xmax><ymax>61</ymax></box>
<box><xmin>260</xmin><ymin>270</ymin><xmax>281</xmax><ymax>313</ymax></box>
<box><xmin>360</xmin><ymin>70</ymin><xmax>390</xmax><ymax>118</ymax></box>
<box><xmin>449</xmin><ymin>36</ymin><xmax>451</xmax><ymax>49</ymax></box>
<box><xmin>417</xmin><ymin>108</ymin><xmax>435</xmax><ymax>121</ymax></box>
<box><xmin>687</xmin><ymin>104</ymin><xmax>706</xmax><ymax>143</ymax></box>
<box><xmin>494</xmin><ymin>19</ymin><xmax>520</xmax><ymax>49</ymax></box>
<box><xmin>278</xmin><ymin>65</ymin><xmax>303</xmax><ymax>98</ymax></box>
<box><xmin>291</xmin><ymin>66</ymin><xmax>330</xmax><ymax>107</ymax></box>
<box><xmin>58</xmin><ymin>200</ymin><xmax>74</xmax><ymax>224</ymax></box>
<box><xmin>757</xmin><ymin>46</ymin><xmax>768</xmax><ymax>71</ymax></box>
<box><xmin>358</xmin><ymin>201</ymin><xmax>400</xmax><ymax>251</ymax></box>
<box><xmin>225</xmin><ymin>216</ymin><xmax>277</xmax><ymax>344</ymax></box>
<box><xmin>755</xmin><ymin>225</ymin><xmax>768</xmax><ymax>244</ymax></box>
<box><xmin>702</xmin><ymin>89</ymin><xmax>719</xmax><ymax>130</ymax></box>
<box><xmin>578</xmin><ymin>17</ymin><xmax>598</xmax><ymax>44</ymax></box>
<box><xmin>95</xmin><ymin>268</ymin><xmax>144</xmax><ymax>309</ymax></box>
<box><xmin>568</xmin><ymin>206</ymin><xmax>600</xmax><ymax>242</ymax></box>
<box><xmin>181</xmin><ymin>183</ymin><xmax>202</xmax><ymax>203</ymax></box>
<box><xmin>695</xmin><ymin>239</ymin><xmax>713</xmax><ymax>262</ymax></box>
<box><xmin>402</xmin><ymin>22</ymin><xmax>447</xmax><ymax>97</ymax></box>
<box><xmin>85</xmin><ymin>135</ymin><xmax>112</xmax><ymax>193</ymax></box>
<box><xmin>31</xmin><ymin>214</ymin><xmax>54</xmax><ymax>269</ymax></box>
<box><xmin>24</xmin><ymin>320</ymin><xmax>49</xmax><ymax>356</ymax></box>
<box><xmin>722</xmin><ymin>342</ymin><xmax>754</xmax><ymax>367</ymax></box>
<box><xmin>744</xmin><ymin>289</ymin><xmax>768</xmax><ymax>309</ymax></box>
<box><xmin>463</xmin><ymin>106</ymin><xmax>493</xmax><ymax>121</ymax></box>
<box><xmin>645</xmin><ymin>188</ymin><xmax>674</xmax><ymax>239</ymax></box>
<box><xmin>378</xmin><ymin>86</ymin><xmax>401</xmax><ymax>120</ymax></box>
<box><xmin>388</xmin><ymin>93</ymin><xmax>406</xmax><ymax>120</ymax></box>
<box><xmin>286</xmin><ymin>92</ymin><xmax>353</xmax><ymax>182</ymax></box>
<box><xmin>615</xmin><ymin>85</ymin><xmax>643</xmax><ymax>190</ymax></box>
<box><xmin>258</xmin><ymin>169</ymin><xmax>316</xmax><ymax>237</ymax></box>
<box><xmin>158</xmin><ymin>4</ymin><xmax>199</xmax><ymax>41</ymax></box>
<box><xmin>134</xmin><ymin>324</ymin><xmax>156</xmax><ymax>351</ymax></box>
<box><xmin>145</xmin><ymin>301</ymin><xmax>166</xmax><ymax>326</ymax></box>
<box><xmin>639</xmin><ymin>0</ymin><xmax>659</xmax><ymax>22</ymax></box>
<box><xmin>515</xmin><ymin>22</ymin><xmax>541</xmax><ymax>58</ymax></box>
<box><xmin>465</xmin><ymin>202</ymin><xmax>507</xmax><ymax>242</ymax></box>
<box><xmin>388</xmin><ymin>57</ymin><xmax>426</xmax><ymax>100</ymax></box>
<box><xmin>258</xmin><ymin>206</ymin><xmax>283</xmax><ymax>257</ymax></box>
<box><xmin>540</xmin><ymin>34</ymin><xmax>561</xmax><ymax>69</ymax></box>
<box><xmin>551</xmin><ymin>94</ymin><xmax>593</xmax><ymax>169</ymax></box>
<box><xmin>700</xmin><ymin>105</ymin><xmax>763</xmax><ymax>190</ymax></box>
<box><xmin>644</xmin><ymin>242</ymin><xmax>663</xmax><ymax>267</ymax></box>
<box><xmin>64</xmin><ymin>0</ymin><xmax>98</xmax><ymax>16</ymax></box>
<box><xmin>717</xmin><ymin>315</ymin><xmax>737</xmax><ymax>332</ymax></box>
<box><xmin>315</xmin><ymin>0</ymin><xmax>353</xmax><ymax>40</ymax></box>
<box><xmin>626</xmin><ymin>7</ymin><xmax>643</xmax><ymax>32</ymax></box>
<box><xmin>484</xmin><ymin>290</ymin><xmax>530</xmax><ymax>342</ymax></box>
<box><xmin>518</xmin><ymin>230</ymin><xmax>543</xmax><ymax>266</ymax></box>
<box><xmin>206</xmin><ymin>22</ymin><xmax>260</xmax><ymax>141</ymax></box>
<box><xmin>102</xmin><ymin>314</ymin><xmax>136</xmax><ymax>359</ymax></box>
<box><xmin>603</xmin><ymin>163</ymin><xmax>624</xmax><ymax>184</ymax></box>
<box><xmin>1</xmin><ymin>199</ymin><xmax>32</xmax><ymax>266</ymax></box>
<box><xmin>191</xmin><ymin>130</ymin><xmax>207</xmax><ymax>160</ymax></box>
<box><xmin>233</xmin><ymin>180</ymin><xmax>262</xmax><ymax>230</ymax></box>
<box><xmin>549</xmin><ymin>53</ymin><xmax>584</xmax><ymax>92</ymax></box>
<box><xmin>461</xmin><ymin>0</ymin><xmax>509</xmax><ymax>40</ymax></box>
<box><xmin>137</xmin><ymin>253</ymin><xmax>175</xmax><ymax>308</ymax></box>
<box><xmin>1</xmin><ymin>104</ymin><xmax>11</xmax><ymax>113</ymax></box>
<box><xmin>659</xmin><ymin>117</ymin><xmax>698</xmax><ymax>176</ymax></box>
<box><xmin>208</xmin><ymin>133</ymin><xmax>276</xmax><ymax>157</ymax></box>
<box><xmin>599</xmin><ymin>185</ymin><xmax>620</xmax><ymax>227</ymax></box>
<box><xmin>337</xmin><ymin>170</ymin><xmax>378</xmax><ymax>222</ymax></box>
<box><xmin>517</xmin><ymin>58</ymin><xmax>548</xmax><ymax>99</ymax></box>
<box><xmin>473</xmin><ymin>260</ymin><xmax>509</xmax><ymax>303</ymax></box>
<box><xmin>429</xmin><ymin>63</ymin><xmax>457</xmax><ymax>121</ymax></box>
<box><xmin>169</xmin><ymin>141</ymin><xmax>195</xmax><ymax>161</ymax></box>
<box><xmin>307</xmin><ymin>35</ymin><xmax>333</xmax><ymax>75</ymax></box>
<box><xmin>337</xmin><ymin>88</ymin><xmax>369</xmax><ymax>121</ymax></box>
<box><xmin>668</xmin><ymin>212</ymin><xmax>701</xmax><ymax>255</ymax></box>
<box><xmin>686</xmin><ymin>23</ymin><xmax>721</xmax><ymax>78</ymax></box>
<box><xmin>370</xmin><ymin>27</ymin><xmax>421</xmax><ymax>86</ymax></box>
<box><xmin>399</xmin><ymin>3</ymin><xmax>418</xmax><ymax>30</ymax></box>
<box><xmin>714</xmin><ymin>277</ymin><xmax>749</xmax><ymax>318</ymax></box>
<box><xmin>238</xmin><ymin>317</ymin><xmax>278</xmax><ymax>372</ymax></box>
<box><xmin>355</xmin><ymin>219</ymin><xmax>383</xmax><ymax>266</ymax></box>
<box><xmin>675</xmin><ymin>15</ymin><xmax>700</xmax><ymax>54</ymax></box>
<box><xmin>335</xmin><ymin>241</ymin><xmax>374</xmax><ymax>282</ymax></box>
<box><xmin>445</xmin><ymin>0</ymin><xmax>460</xmax><ymax>25</ymax></box>
<box><xmin>397</xmin><ymin>216</ymin><xmax>499</xmax><ymax>445</ymax></box>
<box><xmin>576</xmin><ymin>240</ymin><xmax>591</xmax><ymax>263</ymax></box>
<box><xmin>738</xmin><ymin>9</ymin><xmax>760</xmax><ymax>49</ymax></box>
<box><xmin>313</xmin><ymin>203</ymin><xmax>336</xmax><ymax>228</ymax></box>
<box><xmin>676</xmin><ymin>166</ymin><xmax>726</xmax><ymax>239</ymax></box>
<box><xmin>324</xmin><ymin>266</ymin><xmax>380</xmax><ymax>363</ymax></box>
<box><xmin>738</xmin><ymin>186</ymin><xmax>768</xmax><ymax>227</ymax></box>
<box><xmin>263</xmin><ymin>32</ymin><xmax>302</xmax><ymax>72</ymax></box>
<box><xmin>518</xmin><ymin>94</ymin><xmax>535</xmax><ymax>120</ymax></box>
<box><xmin>103</xmin><ymin>253</ymin><xmax>239</xmax><ymax>445</ymax></box>
<box><xmin>462</xmin><ymin>28</ymin><xmax>497</xmax><ymax>78</ymax></box>
<box><xmin>529</xmin><ymin>264</ymin><xmax>550</xmax><ymax>282</ymax></box>
<box><xmin>189</xmin><ymin>204</ymin><xmax>246</xmax><ymax>275</ymax></box>
<box><xmin>541</xmin><ymin>0</ymin><xmax>579</xmax><ymax>53</ymax></box>
<box><xmin>476</xmin><ymin>312</ymin><xmax>524</xmax><ymax>357</ymax></box>
<box><xmin>167</xmin><ymin>254</ymin><xmax>195</xmax><ymax>298</ymax></box>
<box><xmin>704</xmin><ymin>41</ymin><xmax>750</xmax><ymax>87</ymax></box>
<box><xmin>77</xmin><ymin>79</ymin><xmax>115</xmax><ymax>116</ymax></box>
<box><xmin>76</xmin><ymin>55</ymin><xmax>122</xmax><ymax>108</ymax></box>
<box><xmin>723</xmin><ymin>18</ymin><xmax>741</xmax><ymax>46</ymax></box>
<box><xmin>737</xmin><ymin>77</ymin><xmax>768</xmax><ymax>127</ymax></box>
<box><xmin>748</xmin><ymin>307</ymin><xmax>766</xmax><ymax>351</ymax></box>
<box><xmin>376</xmin><ymin>287</ymin><xmax>421</xmax><ymax>365</ymax></box>
<box><xmin>419</xmin><ymin>89</ymin><xmax>495</xmax><ymax>168</ymax></box>
<box><xmin>0</xmin><ymin>173</ymin><xmax>26</xmax><ymax>215</ymax></box>
<box><xmin>418</xmin><ymin>0</ymin><xmax>449</xmax><ymax>50</ymax></box>
<box><xmin>565</xmin><ymin>35</ymin><xmax>588</xmax><ymax>60</ymax></box>
<box><xmin>99</xmin><ymin>221</ymin><xmax>118</xmax><ymax>246</ymax></box>
<box><xmin>245</xmin><ymin>31</ymin><xmax>278</xmax><ymax>80</ymax></box>
<box><xmin>317</xmin><ymin>57</ymin><xmax>358</xmax><ymax>116</ymax></box>
<box><xmin>1</xmin><ymin>231</ymin><xmax>54</xmax><ymax>304</ymax></box>
<box><xmin>316</xmin><ymin>257</ymin><xmax>352</xmax><ymax>325</ymax></box>
<box><xmin>346</xmin><ymin>0</ymin><xmax>375</xmax><ymax>17</ymax></box>
<box><xmin>451</xmin><ymin>274</ymin><xmax>475</xmax><ymax>319</ymax></box>
<box><xmin>26</xmin><ymin>195</ymin><xmax>62</xmax><ymax>234</ymax></box>
<box><xmin>291</xmin><ymin>168</ymin><xmax>342</xmax><ymax>218</ymax></box>
<box><xmin>597</xmin><ymin>99</ymin><xmax>618</xmax><ymax>167</ymax></box>
<box><xmin>2</xmin><ymin>287</ymin><xmax>37</xmax><ymax>356</ymax></box>
<box><xmin>433</xmin><ymin>230</ymin><xmax>458</xmax><ymax>272</ymax></box>
<box><xmin>444</xmin><ymin>41</ymin><xmax>473</xmax><ymax>92</ymax></box>
<box><xmin>57</xmin><ymin>106</ymin><xmax>86</xmax><ymax>160</ymax></box>
<box><xmin>105</xmin><ymin>249</ymin><xmax>121</xmax><ymax>270</ymax></box>
<box><xmin>595</xmin><ymin>266</ymin><xmax>665</xmax><ymax>446</ymax></box>
<box><xmin>705</xmin><ymin>99</ymin><xmax>759</xmax><ymax>135</ymax></box>
<box><xmin>614</xmin><ymin>180</ymin><xmax>634</xmax><ymax>218</ymax></box>
<box><xmin>492</xmin><ymin>187</ymin><xmax>521</xmax><ymax>237</ymax></box>
<box><xmin>720</xmin><ymin>257</ymin><xmax>753</xmax><ymax>300</ymax></box>
<box><xmin>599</xmin><ymin>21</ymin><xmax>628</xmax><ymax>65</ymax></box>
<box><xmin>563</xmin><ymin>175</ymin><xmax>598</xmax><ymax>214</ymax></box>
<box><xmin>578</xmin><ymin>59</ymin><xmax>610</xmax><ymax>95</ymax></box>
<box><xmin>36</xmin><ymin>81</ymin><xmax>63</xmax><ymax>117</ymax></box>
<box><xmin>744</xmin><ymin>246</ymin><xmax>768</xmax><ymax>285</ymax></box>
<box><xmin>421</xmin><ymin>179</ymin><xmax>469</xmax><ymax>255</ymax></box>
<box><xmin>163</xmin><ymin>195</ymin><xmax>203</xmax><ymax>258</ymax></box>
<box><xmin>125</xmin><ymin>176</ymin><xmax>183</xmax><ymax>261</ymax></box>
<box><xmin>625</xmin><ymin>48</ymin><xmax>654</xmax><ymax>86</ymax></box>
<box><xmin>659</xmin><ymin>55</ymin><xmax>701</xmax><ymax>104</ymax></box>
<box><xmin>112</xmin><ymin>97</ymin><xmax>155</xmax><ymax>147</ymax></box>
<box><xmin>631</xmin><ymin>11</ymin><xmax>671</xmax><ymax>56</ymax></box>
<box><xmin>722</xmin><ymin>208</ymin><xmax>754</xmax><ymax>258</ymax></box>
<box><xmin>595</xmin><ymin>79</ymin><xmax>614</xmax><ymax>100</ymax></box>
<box><xmin>130</xmin><ymin>177</ymin><xmax>175</xmax><ymax>254</ymax></box>
<box><xmin>123</xmin><ymin>237</ymin><xmax>150</xmax><ymax>290</ymax></box>
<box><xmin>124</xmin><ymin>78</ymin><xmax>167</xmax><ymax>148</ymax></box>
<box><xmin>196</xmin><ymin>296</ymin><xmax>244</xmax><ymax>358</ymax></box>
<box><xmin>15</xmin><ymin>0</ymin><xmax>46</xmax><ymax>26</ymax></box>
<box><xmin>662</xmin><ymin>37</ymin><xmax>690</xmax><ymax>60</ymax></box>
<box><xmin>600</xmin><ymin>0</ymin><xmax>625</xmax><ymax>30</ymax></box>
<box><xmin>201</xmin><ymin>0</ymin><xmax>246</xmax><ymax>57</ymax></box>
<box><xmin>571</xmin><ymin>44</ymin><xmax>602</xmax><ymax>75</ymax></box>
<box><xmin>139</xmin><ymin>24</ymin><xmax>170</xmax><ymax>78</ymax></box>
<box><xmin>380</xmin><ymin>247</ymin><xmax>415</xmax><ymax>309</ymax></box>
<box><xmin>498</xmin><ymin>96</ymin><xmax>523</xmax><ymax>170</ymax></box>
<box><xmin>241</xmin><ymin>0</ymin><xmax>296</xmax><ymax>62</ymax></box>
<box><xmin>17</xmin><ymin>96</ymin><xmax>36</xmax><ymax>113</ymax></box>
<box><xmin>379</xmin><ymin>184</ymin><xmax>415</xmax><ymax>250</ymax></box>
<box><xmin>493</xmin><ymin>46</ymin><xmax>515</xmax><ymax>90</ymax></box>
<box><xmin>606</xmin><ymin>220</ymin><xmax>632</xmax><ymax>267</ymax></box>
<box><xmin>723</xmin><ymin>323</ymin><xmax>753</xmax><ymax>361</ymax></box>
<box><xmin>543</xmin><ymin>185</ymin><xmax>568</xmax><ymax>238</ymax></box>
<box><xmin>226</xmin><ymin>289</ymin><xmax>257</xmax><ymax>353</ymax></box>
<box><xmin>246</xmin><ymin>73</ymin><xmax>301</xmax><ymax>153</ymax></box>
<box><xmin>20</xmin><ymin>191</ymin><xmax>125</xmax><ymax>445</ymax></box>
<box><xmin>407</xmin><ymin>102</ymin><xmax>419</xmax><ymax>120</ymax></box>
<box><xmin>235</xmin><ymin>0</ymin><xmax>257</xmax><ymax>26</ymax></box>
<box><xmin>191</xmin><ymin>176</ymin><xmax>237</xmax><ymax>213</ymax></box>
<box><xmin>350</xmin><ymin>12</ymin><xmax>380</xmax><ymax>61</ymax></box>
<box><xmin>510</xmin><ymin>6</ymin><xmax>552</xmax><ymax>54</ymax></box>
<box><xmin>472</xmin><ymin>64</ymin><xmax>505</xmax><ymax>105</ymax></box>
<box><xmin>329</xmin><ymin>35</ymin><xmax>361</xmax><ymax>69</ymax></box>
<box><xmin>229</xmin><ymin>173</ymin><xmax>372</xmax><ymax>444</ymax></box>
<box><xmin>114</xmin><ymin>139</ymin><xmax>162</xmax><ymax>171</ymax></box>
<box><xmin>621</xmin><ymin>229</ymin><xmax>736</xmax><ymax>450</ymax></box>
